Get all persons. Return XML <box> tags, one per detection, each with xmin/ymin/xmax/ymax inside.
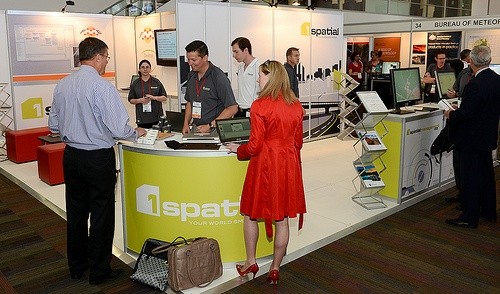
<box><xmin>128</xmin><ymin>60</ymin><xmax>167</xmax><ymax>129</ymax></box>
<box><xmin>364</xmin><ymin>50</ymin><xmax>384</xmax><ymax>91</ymax></box>
<box><xmin>49</xmin><ymin>37</ymin><xmax>147</xmax><ymax>284</ymax></box>
<box><xmin>347</xmin><ymin>48</ymin><xmax>352</xmax><ymax>64</ymax></box>
<box><xmin>182</xmin><ymin>40</ymin><xmax>239</xmax><ymax>136</ymax></box>
<box><xmin>225</xmin><ymin>57</ymin><xmax>307</xmax><ymax>291</ymax></box>
<box><xmin>412</xmin><ymin>56</ymin><xmax>425</xmax><ymax>64</ymax></box>
<box><xmin>284</xmin><ymin>47</ymin><xmax>300</xmax><ymax>98</ymax></box>
<box><xmin>348</xmin><ymin>52</ymin><xmax>363</xmax><ymax>91</ymax></box>
<box><xmin>445</xmin><ymin>45</ymin><xmax>500</xmax><ymax>228</ymax></box>
<box><xmin>447</xmin><ymin>38</ymin><xmax>487</xmax><ymax>98</ymax></box>
<box><xmin>228</xmin><ymin>37</ymin><xmax>263</xmax><ymax>118</ymax></box>
<box><xmin>423</xmin><ymin>50</ymin><xmax>450</xmax><ymax>103</ymax></box>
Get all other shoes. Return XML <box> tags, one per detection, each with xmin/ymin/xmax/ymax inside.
<box><xmin>445</xmin><ymin>196</ymin><xmax>460</xmax><ymax>203</ymax></box>
<box><xmin>91</xmin><ymin>268</ymin><xmax>125</xmax><ymax>285</ymax></box>
<box><xmin>480</xmin><ymin>212</ymin><xmax>497</xmax><ymax>223</ymax></box>
<box><xmin>456</xmin><ymin>205</ymin><xmax>464</xmax><ymax>211</ymax></box>
<box><xmin>70</xmin><ymin>263</ymin><xmax>89</xmax><ymax>280</ymax></box>
<box><xmin>445</xmin><ymin>218</ymin><xmax>477</xmax><ymax>229</ymax></box>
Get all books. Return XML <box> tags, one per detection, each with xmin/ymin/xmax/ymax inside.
<box><xmin>353</xmin><ymin>162</ymin><xmax>386</xmax><ymax>187</ymax></box>
<box><xmin>438</xmin><ymin>99</ymin><xmax>455</xmax><ymax>114</ymax></box>
<box><xmin>358</xmin><ymin>131</ymin><xmax>387</xmax><ymax>152</ymax></box>
<box><xmin>138</xmin><ymin>129</ymin><xmax>159</xmax><ymax>145</ymax></box>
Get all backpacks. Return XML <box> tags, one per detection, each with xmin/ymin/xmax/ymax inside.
<box><xmin>452</xmin><ymin>64</ymin><xmax>474</xmax><ymax>100</ymax></box>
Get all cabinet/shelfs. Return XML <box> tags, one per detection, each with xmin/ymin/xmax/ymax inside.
<box><xmin>351</xmin><ymin>90</ymin><xmax>391</xmax><ymax>210</ymax></box>
<box><xmin>0</xmin><ymin>83</ymin><xmax>15</xmax><ymax>162</ymax></box>
<box><xmin>329</xmin><ymin>72</ymin><xmax>361</xmax><ymax>141</ymax></box>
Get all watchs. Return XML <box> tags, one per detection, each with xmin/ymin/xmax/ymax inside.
<box><xmin>209</xmin><ymin>122</ymin><xmax>215</xmax><ymax>131</ymax></box>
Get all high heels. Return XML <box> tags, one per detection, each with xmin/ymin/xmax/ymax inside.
<box><xmin>236</xmin><ymin>263</ymin><xmax>259</xmax><ymax>280</ymax></box>
<box><xmin>268</xmin><ymin>270</ymin><xmax>278</xmax><ymax>287</ymax></box>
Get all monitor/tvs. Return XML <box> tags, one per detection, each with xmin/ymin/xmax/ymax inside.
<box><xmin>490</xmin><ymin>64</ymin><xmax>500</xmax><ymax>75</ymax></box>
<box><xmin>434</xmin><ymin>71</ymin><xmax>457</xmax><ymax>99</ymax></box>
<box><xmin>381</xmin><ymin>62</ymin><xmax>422</xmax><ymax>106</ymax></box>
<box><xmin>155</xmin><ymin>29</ymin><xmax>177</xmax><ymax>67</ymax></box>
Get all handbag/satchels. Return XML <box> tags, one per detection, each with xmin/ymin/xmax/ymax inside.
<box><xmin>129</xmin><ymin>238</ymin><xmax>178</xmax><ymax>292</ymax></box>
<box><xmin>151</xmin><ymin>235</ymin><xmax>223</xmax><ymax>291</ymax></box>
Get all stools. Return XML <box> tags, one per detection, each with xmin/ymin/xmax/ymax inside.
<box><xmin>36</xmin><ymin>142</ymin><xmax>67</xmax><ymax>187</ymax></box>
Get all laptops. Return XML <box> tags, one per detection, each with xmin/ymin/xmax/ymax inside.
<box><xmin>215</xmin><ymin>117</ymin><xmax>251</xmax><ymax>144</ymax></box>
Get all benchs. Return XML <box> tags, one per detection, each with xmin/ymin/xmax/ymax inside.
<box><xmin>5</xmin><ymin>124</ymin><xmax>52</xmax><ymax>164</ymax></box>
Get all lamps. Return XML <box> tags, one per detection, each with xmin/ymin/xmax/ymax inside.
<box><xmin>61</xmin><ymin>0</ymin><xmax>75</xmax><ymax>13</ymax></box>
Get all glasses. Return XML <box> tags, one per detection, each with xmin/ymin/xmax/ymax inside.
<box><xmin>264</xmin><ymin>59</ymin><xmax>270</xmax><ymax>72</ymax></box>
<box><xmin>95</xmin><ymin>53</ymin><xmax>110</xmax><ymax>61</ymax></box>
<box><xmin>438</xmin><ymin>57</ymin><xmax>445</xmax><ymax>60</ymax></box>
